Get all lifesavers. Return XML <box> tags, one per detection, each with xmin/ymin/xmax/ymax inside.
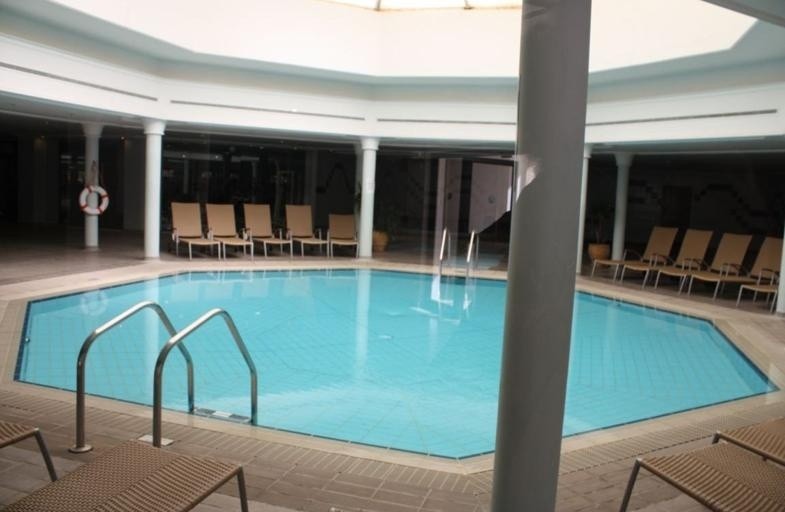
<box><xmin>80</xmin><ymin>186</ymin><xmax>108</xmax><ymax>215</ymax></box>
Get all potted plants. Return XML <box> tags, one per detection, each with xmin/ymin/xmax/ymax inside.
<box><xmin>373</xmin><ymin>204</ymin><xmax>401</xmax><ymax>253</ymax></box>
<box><xmin>588</xmin><ymin>215</ymin><xmax>611</xmax><ymax>268</ymax></box>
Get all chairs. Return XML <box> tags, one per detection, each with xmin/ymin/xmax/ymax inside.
<box><xmin>0</xmin><ymin>421</ymin><xmax>57</xmax><ymax>482</ymax></box>
<box><xmin>0</xmin><ymin>438</ymin><xmax>248</xmax><ymax>512</ymax></box>
<box><xmin>171</xmin><ymin>202</ymin><xmax>359</xmax><ymax>259</ymax></box>
<box><xmin>621</xmin><ymin>442</ymin><xmax>785</xmax><ymax>512</ymax></box>
<box><xmin>590</xmin><ymin>226</ymin><xmax>783</xmax><ymax>312</ymax></box>
<box><xmin>711</xmin><ymin>420</ymin><xmax>785</xmax><ymax>467</ymax></box>
